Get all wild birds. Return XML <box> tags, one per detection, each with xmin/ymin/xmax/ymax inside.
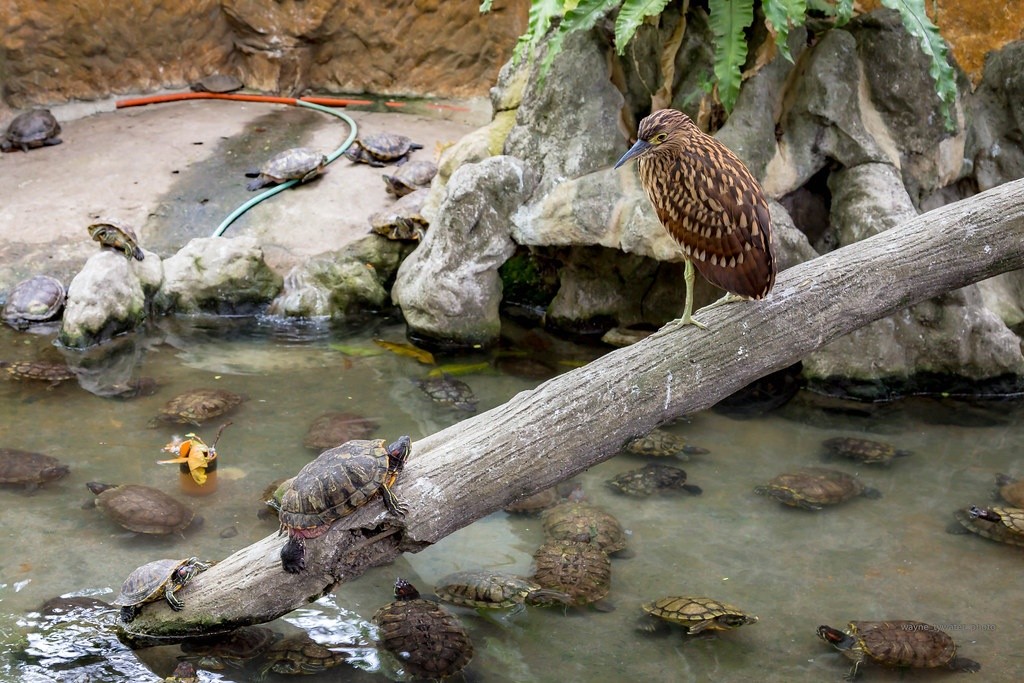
<box><xmin>613</xmin><ymin>108</ymin><xmax>779</xmax><ymax>341</ymax></box>
